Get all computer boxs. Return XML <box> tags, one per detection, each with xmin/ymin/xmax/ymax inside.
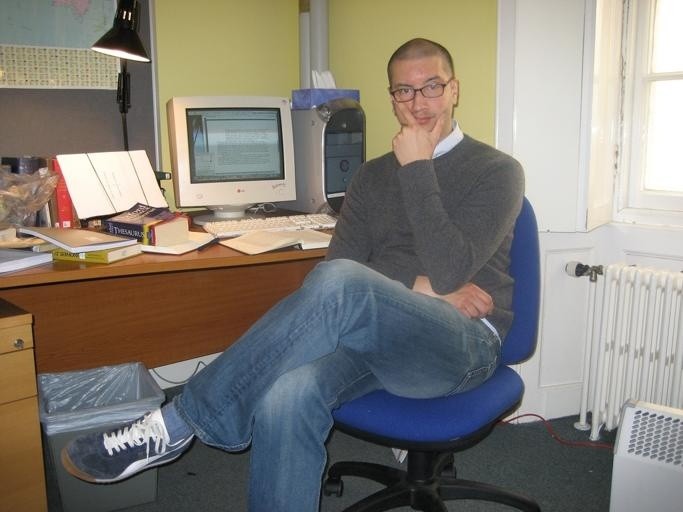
<box><xmin>266</xmin><ymin>97</ymin><xmax>366</xmax><ymax>216</ymax></box>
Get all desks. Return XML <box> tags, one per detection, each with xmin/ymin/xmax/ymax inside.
<box><xmin>0</xmin><ymin>209</ymin><xmax>343</xmax><ymax>369</ymax></box>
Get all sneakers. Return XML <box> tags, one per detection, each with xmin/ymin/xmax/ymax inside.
<box><xmin>59</xmin><ymin>407</ymin><xmax>195</xmax><ymax>484</ymax></box>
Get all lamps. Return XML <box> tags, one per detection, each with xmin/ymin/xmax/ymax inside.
<box><xmin>91</xmin><ymin>0</ymin><xmax>151</xmax><ymax>151</ymax></box>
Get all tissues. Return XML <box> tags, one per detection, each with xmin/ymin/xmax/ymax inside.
<box><xmin>291</xmin><ymin>70</ymin><xmax>361</xmax><ymax>108</ymax></box>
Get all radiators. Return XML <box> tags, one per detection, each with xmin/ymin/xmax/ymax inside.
<box><xmin>569</xmin><ymin>260</ymin><xmax>683</xmax><ymax>444</ymax></box>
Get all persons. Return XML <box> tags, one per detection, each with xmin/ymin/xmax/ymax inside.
<box><xmin>59</xmin><ymin>38</ymin><xmax>526</xmax><ymax>512</ymax></box>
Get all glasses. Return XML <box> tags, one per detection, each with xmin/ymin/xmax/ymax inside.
<box><xmin>389</xmin><ymin>77</ymin><xmax>455</xmax><ymax>102</ymax></box>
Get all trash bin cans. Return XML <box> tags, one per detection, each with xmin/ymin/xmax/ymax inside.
<box><xmin>35</xmin><ymin>360</ymin><xmax>166</xmax><ymax>512</ymax></box>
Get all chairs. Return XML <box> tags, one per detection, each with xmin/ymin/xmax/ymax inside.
<box><xmin>322</xmin><ymin>194</ymin><xmax>541</xmax><ymax>512</ymax></box>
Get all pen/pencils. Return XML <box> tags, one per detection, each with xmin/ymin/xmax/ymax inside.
<box><xmin>198</xmin><ymin>237</ymin><xmax>220</xmax><ymax>251</ymax></box>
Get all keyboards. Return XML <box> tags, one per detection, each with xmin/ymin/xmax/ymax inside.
<box><xmin>203</xmin><ymin>213</ymin><xmax>338</xmax><ymax>239</ymax></box>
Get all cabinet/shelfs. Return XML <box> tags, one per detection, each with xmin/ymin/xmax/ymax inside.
<box><xmin>1</xmin><ymin>294</ymin><xmax>48</xmax><ymax>512</ymax></box>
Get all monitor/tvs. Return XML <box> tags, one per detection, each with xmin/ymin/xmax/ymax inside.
<box><xmin>166</xmin><ymin>96</ymin><xmax>297</xmax><ymax>226</ymax></box>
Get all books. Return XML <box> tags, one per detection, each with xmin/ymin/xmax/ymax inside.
<box><xmin>18</xmin><ymin>203</ymin><xmax>190</xmax><ymax>265</ymax></box>
<box><xmin>217</xmin><ymin>227</ymin><xmax>333</xmax><ymax>256</ymax></box>
